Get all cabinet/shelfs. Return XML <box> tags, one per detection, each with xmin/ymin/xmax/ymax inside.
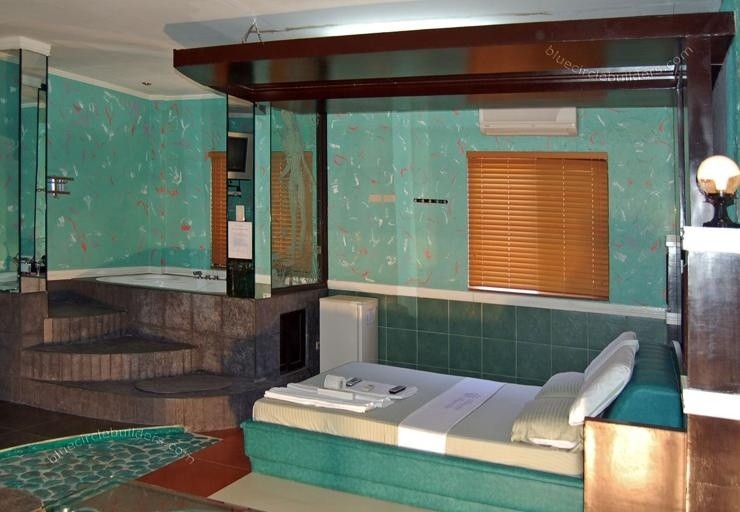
<box><xmin>583</xmin><ymin>415</ymin><xmax>740</xmax><ymax>512</ymax></box>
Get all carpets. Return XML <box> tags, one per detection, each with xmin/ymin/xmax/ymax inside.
<box><xmin>132</xmin><ymin>374</ymin><xmax>234</xmax><ymax>394</ymax></box>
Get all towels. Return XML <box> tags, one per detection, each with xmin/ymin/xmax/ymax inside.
<box><xmin>264</xmin><ymin>377</ymin><xmax>417</xmax><ymax>414</ymax></box>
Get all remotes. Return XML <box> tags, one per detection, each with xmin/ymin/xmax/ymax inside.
<box><xmin>389</xmin><ymin>385</ymin><xmax>406</xmax><ymax>394</ymax></box>
<box><xmin>346</xmin><ymin>377</ymin><xmax>361</xmax><ymax>387</ymax></box>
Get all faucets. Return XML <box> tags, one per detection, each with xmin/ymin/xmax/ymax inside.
<box><xmin>193</xmin><ymin>271</ymin><xmax>202</xmax><ymax>278</ymax></box>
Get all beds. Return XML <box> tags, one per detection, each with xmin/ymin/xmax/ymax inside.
<box><xmin>242</xmin><ymin>341</ymin><xmax>688</xmax><ymax>512</ymax></box>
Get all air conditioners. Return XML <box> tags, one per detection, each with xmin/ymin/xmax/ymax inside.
<box><xmin>479</xmin><ymin>106</ymin><xmax>579</xmax><ymax>136</ymax></box>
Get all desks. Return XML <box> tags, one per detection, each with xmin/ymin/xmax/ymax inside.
<box><xmin>34</xmin><ymin>475</ymin><xmax>264</xmax><ymax>512</ymax></box>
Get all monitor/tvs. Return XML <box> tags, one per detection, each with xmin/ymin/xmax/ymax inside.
<box><xmin>227</xmin><ymin>131</ymin><xmax>254</xmax><ymax>180</ymax></box>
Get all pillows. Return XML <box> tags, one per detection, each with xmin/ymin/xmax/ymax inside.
<box><xmin>535</xmin><ymin>371</ymin><xmax>585</xmax><ymax>399</ymax></box>
<box><xmin>584</xmin><ymin>330</ymin><xmax>639</xmax><ymax>381</ymax></box>
<box><xmin>568</xmin><ymin>345</ymin><xmax>637</xmax><ymax>426</ymax></box>
<box><xmin>511</xmin><ymin>398</ymin><xmax>604</xmax><ymax>451</ymax></box>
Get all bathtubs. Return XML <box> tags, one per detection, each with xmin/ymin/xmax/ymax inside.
<box><xmin>96</xmin><ymin>274</ymin><xmax>226</xmax><ymax>293</ymax></box>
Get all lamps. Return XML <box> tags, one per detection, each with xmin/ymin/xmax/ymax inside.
<box><xmin>695</xmin><ymin>154</ymin><xmax>740</xmax><ymax>228</ymax></box>
